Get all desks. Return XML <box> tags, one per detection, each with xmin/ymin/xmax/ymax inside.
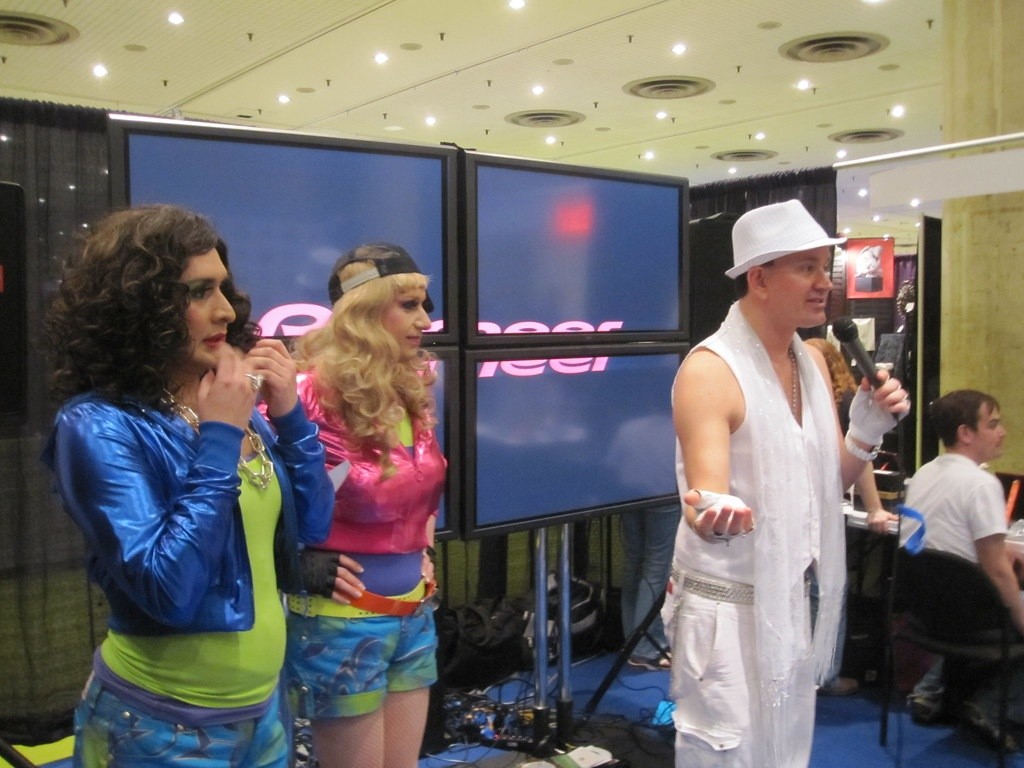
<box><xmin>841</xmin><ymin>497</ymin><xmax>1023</xmax><ymax>679</ymax></box>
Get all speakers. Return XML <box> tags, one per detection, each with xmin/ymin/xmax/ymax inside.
<box><xmin>0</xmin><ymin>181</ymin><xmax>30</xmax><ymax>427</ymax></box>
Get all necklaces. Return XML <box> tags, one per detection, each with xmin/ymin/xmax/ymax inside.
<box><xmin>159</xmin><ymin>389</ymin><xmax>274</xmax><ymax>490</ymax></box>
<box><xmin>790</xmin><ymin>350</ymin><xmax>797</xmax><ymax>414</ymax></box>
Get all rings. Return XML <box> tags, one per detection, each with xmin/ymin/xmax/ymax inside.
<box><xmin>244</xmin><ymin>373</ymin><xmax>264</xmax><ymax>391</ymax></box>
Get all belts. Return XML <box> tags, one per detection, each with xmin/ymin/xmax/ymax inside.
<box><xmin>337</xmin><ymin>579</ymin><xmax>437</xmax><ymax>618</ymax></box>
<box><xmin>282</xmin><ymin>578</ymin><xmax>425</xmax><ymax>618</ymax></box>
<box><xmin>670</xmin><ymin>568</ymin><xmax>812</xmax><ymax>606</ymax></box>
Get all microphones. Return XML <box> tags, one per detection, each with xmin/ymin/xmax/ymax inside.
<box><xmin>833</xmin><ymin>317</ymin><xmax>900</xmax><ymax>422</ymax></box>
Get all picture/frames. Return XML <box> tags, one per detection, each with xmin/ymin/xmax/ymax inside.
<box><xmin>844</xmin><ymin>236</ymin><xmax>898</xmax><ymax>300</ymax></box>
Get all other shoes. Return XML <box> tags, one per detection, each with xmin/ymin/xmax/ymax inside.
<box><xmin>820</xmin><ymin>675</ymin><xmax>862</xmax><ymax>695</ymax></box>
<box><xmin>629</xmin><ymin>650</ymin><xmax>672</xmax><ymax>672</ymax></box>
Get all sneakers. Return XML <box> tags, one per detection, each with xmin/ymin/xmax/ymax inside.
<box><xmin>909</xmin><ymin>696</ymin><xmax>966</xmax><ymax>724</ymax></box>
<box><xmin>968</xmin><ymin>693</ymin><xmax>1020</xmax><ymax>753</ymax></box>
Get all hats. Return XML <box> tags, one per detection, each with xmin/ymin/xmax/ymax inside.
<box><xmin>724</xmin><ymin>199</ymin><xmax>848</xmax><ymax>280</ymax></box>
<box><xmin>327</xmin><ymin>243</ymin><xmax>434</xmax><ymax>313</ymax></box>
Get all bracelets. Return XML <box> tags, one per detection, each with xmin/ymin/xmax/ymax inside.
<box><xmin>426</xmin><ymin>546</ymin><xmax>437</xmax><ymax>564</ymax></box>
<box><xmin>845</xmin><ymin>430</ymin><xmax>882</xmax><ymax>462</ymax></box>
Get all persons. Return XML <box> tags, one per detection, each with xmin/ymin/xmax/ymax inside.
<box><xmin>806</xmin><ymin>338</ymin><xmax>898</xmax><ymax>696</ymax></box>
<box><xmin>258</xmin><ymin>241</ymin><xmax>446</xmax><ymax>768</ymax></box>
<box><xmin>51</xmin><ymin>206</ymin><xmax>335</xmax><ymax>768</ymax></box>
<box><xmin>899</xmin><ymin>389</ymin><xmax>1024</xmax><ymax>752</ymax></box>
<box><xmin>621</xmin><ymin>503</ymin><xmax>682</xmax><ymax>670</ymax></box>
<box><xmin>661</xmin><ymin>199</ymin><xmax>910</xmax><ymax>768</ymax></box>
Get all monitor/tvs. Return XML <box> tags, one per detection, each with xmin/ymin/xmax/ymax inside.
<box><xmin>104</xmin><ymin>112</ymin><xmax>693</xmax><ymax>542</ymax></box>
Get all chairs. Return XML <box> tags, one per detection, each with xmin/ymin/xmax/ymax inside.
<box><xmin>877</xmin><ymin>546</ymin><xmax>1024</xmax><ymax>767</ymax></box>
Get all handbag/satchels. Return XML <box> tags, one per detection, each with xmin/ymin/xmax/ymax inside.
<box><xmin>522</xmin><ymin>568</ymin><xmax>602</xmax><ymax>666</ymax></box>
<box><xmin>434</xmin><ymin>592</ymin><xmax>524</xmax><ymax>689</ymax></box>
